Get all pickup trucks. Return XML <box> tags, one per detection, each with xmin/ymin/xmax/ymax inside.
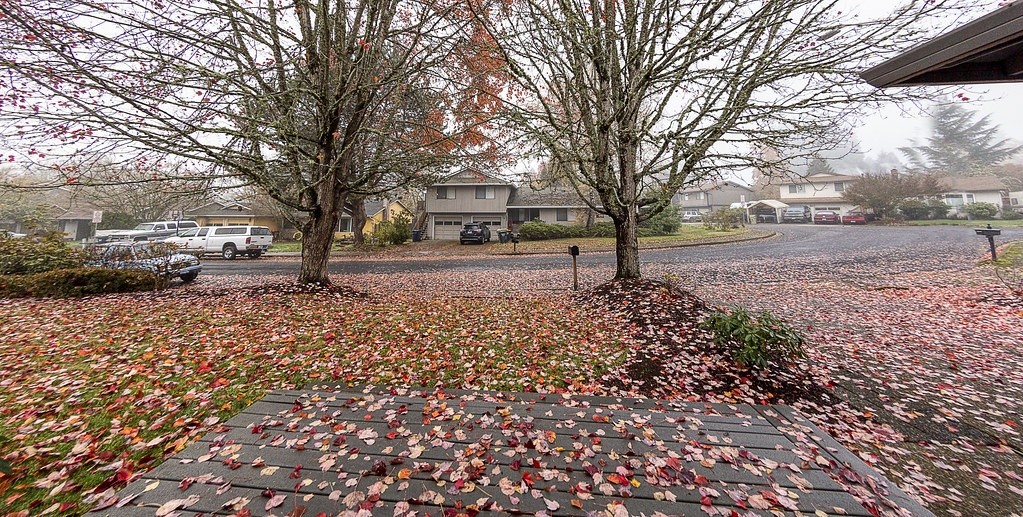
<box><xmin>164</xmin><ymin>226</ymin><xmax>273</xmax><ymax>260</ymax></box>
<box><xmin>123</xmin><ymin>220</ymin><xmax>200</xmax><ymax>246</ymax></box>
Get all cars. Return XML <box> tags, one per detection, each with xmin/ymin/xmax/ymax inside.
<box><xmin>7</xmin><ymin>231</ymin><xmax>27</xmax><ymax>240</ymax></box>
<box><xmin>842</xmin><ymin>210</ymin><xmax>868</xmax><ymax>225</ymax></box>
<box><xmin>814</xmin><ymin>210</ymin><xmax>841</xmax><ymax>224</ymax></box>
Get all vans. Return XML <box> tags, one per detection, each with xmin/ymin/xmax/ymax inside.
<box><xmin>681</xmin><ymin>211</ymin><xmax>703</xmax><ymax>223</ymax></box>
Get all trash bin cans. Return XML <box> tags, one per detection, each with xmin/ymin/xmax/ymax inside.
<box><xmin>412</xmin><ymin>230</ymin><xmax>421</xmax><ymax>242</ymax></box>
<box><xmin>496</xmin><ymin>228</ymin><xmax>511</xmax><ymax>243</ymax></box>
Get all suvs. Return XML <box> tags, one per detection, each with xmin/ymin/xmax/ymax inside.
<box><xmin>83</xmin><ymin>241</ymin><xmax>203</xmax><ymax>282</ymax></box>
<box><xmin>459</xmin><ymin>222</ymin><xmax>491</xmax><ymax>245</ymax></box>
<box><xmin>783</xmin><ymin>205</ymin><xmax>812</xmax><ymax>223</ymax></box>
